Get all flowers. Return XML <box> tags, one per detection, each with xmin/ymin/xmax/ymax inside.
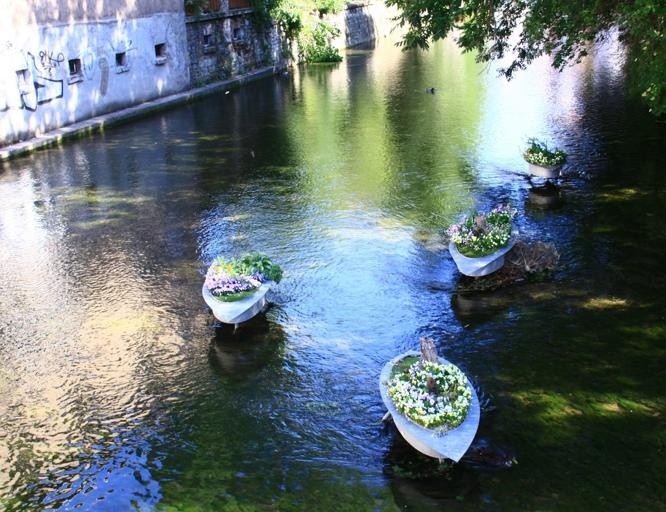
<box><xmin>379</xmin><ymin>354</ymin><xmax>471</xmax><ymax>430</ymax></box>
<box><xmin>516</xmin><ymin>134</ymin><xmax>565</xmax><ymax>167</ymax></box>
<box><xmin>451</xmin><ymin>205</ymin><xmax>516</xmax><ymax>255</ymax></box>
<box><xmin>206</xmin><ymin>254</ymin><xmax>284</xmax><ymax>300</ymax></box>
